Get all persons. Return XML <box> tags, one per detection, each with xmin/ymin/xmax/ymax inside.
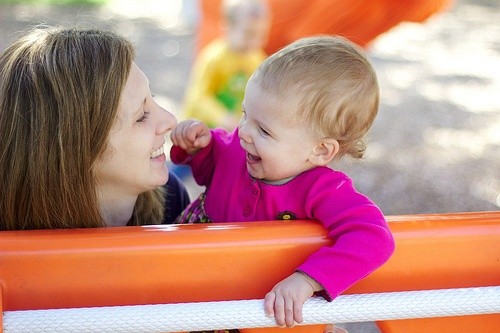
<box><xmin>1</xmin><ymin>26</ymin><xmax>191</xmax><ymax>227</ymax></box>
<box><xmin>164</xmin><ymin>0</ymin><xmax>270</xmax><ymax>185</ymax></box>
<box><xmin>169</xmin><ymin>36</ymin><xmax>396</xmax><ymax>329</ymax></box>
